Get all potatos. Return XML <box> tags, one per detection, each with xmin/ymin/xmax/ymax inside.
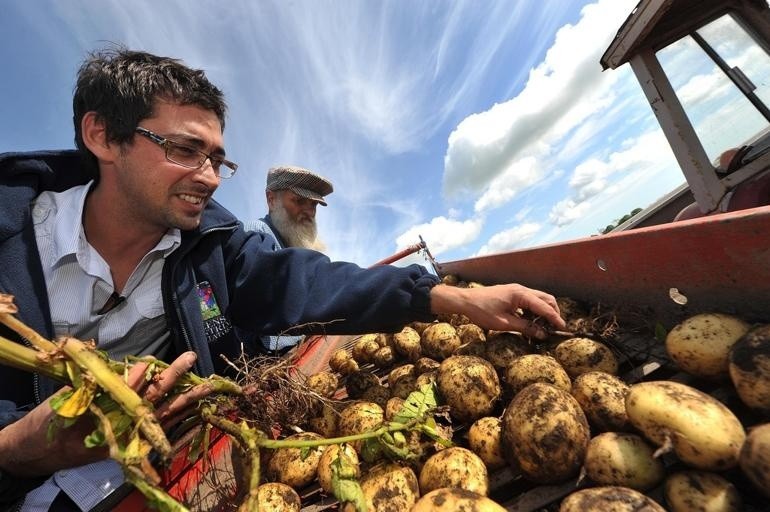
<box><xmin>236</xmin><ymin>275</ymin><xmax>769</xmax><ymax>512</ymax></box>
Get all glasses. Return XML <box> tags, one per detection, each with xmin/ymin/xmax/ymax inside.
<box><xmin>131</xmin><ymin>122</ymin><xmax>238</xmax><ymax>183</ymax></box>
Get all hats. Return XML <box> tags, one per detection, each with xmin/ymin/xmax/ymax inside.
<box><xmin>264</xmin><ymin>163</ymin><xmax>336</xmax><ymax>208</ymax></box>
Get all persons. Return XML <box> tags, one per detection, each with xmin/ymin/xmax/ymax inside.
<box><xmin>1</xmin><ymin>49</ymin><xmax>567</xmax><ymax>511</ymax></box>
<box><xmin>247</xmin><ymin>164</ymin><xmax>334</xmax><ymax>359</ymax></box>
